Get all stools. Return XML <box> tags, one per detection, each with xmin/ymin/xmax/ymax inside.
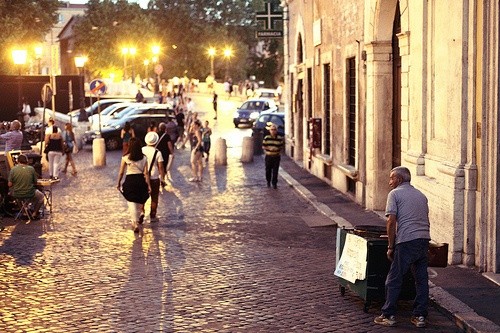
<box><xmin>14</xmin><ymin>198</ymin><xmax>33</xmax><ymax>221</ymax></box>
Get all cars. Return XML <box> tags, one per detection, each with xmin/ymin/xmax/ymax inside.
<box><xmin>109</xmin><ymin>106</ymin><xmax>176</xmax><ymax>127</ymax></box>
<box><xmin>81</xmin><ymin>114</ymin><xmax>179</xmax><ymax>150</ymax></box>
<box><xmin>249</xmin><ymin>88</ymin><xmax>279</xmax><ymax>113</ymax></box>
<box><xmin>252</xmin><ymin>114</ymin><xmax>284</xmax><ymax>138</ymax></box>
<box><xmin>232</xmin><ymin>98</ymin><xmax>277</xmax><ymax>128</ymax></box>
<box><xmin>88</xmin><ymin>103</ymin><xmax>129</xmax><ymax>124</ymax></box>
<box><xmin>67</xmin><ymin>100</ymin><xmax>126</xmax><ymax>120</ymax></box>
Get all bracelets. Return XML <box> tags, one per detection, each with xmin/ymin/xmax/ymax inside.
<box><xmin>387</xmin><ymin>245</ymin><xmax>393</xmax><ymax>249</ymax></box>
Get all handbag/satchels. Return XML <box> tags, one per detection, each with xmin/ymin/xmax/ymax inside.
<box><xmin>63</xmin><ymin>141</ymin><xmax>68</xmax><ymax>153</ymax></box>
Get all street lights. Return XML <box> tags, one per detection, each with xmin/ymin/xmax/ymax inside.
<box><xmin>152</xmin><ymin>46</ymin><xmax>161</xmax><ymax>84</ymax></box>
<box><xmin>143</xmin><ymin>59</ymin><xmax>149</xmax><ymax>82</ymax></box>
<box><xmin>121</xmin><ymin>47</ymin><xmax>128</xmax><ymax>80</ymax></box>
<box><xmin>129</xmin><ymin>47</ymin><xmax>136</xmax><ymax>83</ymax></box>
<box><xmin>209</xmin><ymin>47</ymin><xmax>215</xmax><ymax>76</ymax></box>
<box><xmin>11</xmin><ymin>48</ymin><xmax>28</xmax><ymax>130</ymax></box>
<box><xmin>34</xmin><ymin>46</ymin><xmax>44</xmax><ymax>76</ymax></box>
<box><xmin>74</xmin><ymin>54</ymin><xmax>85</xmax><ymax>76</ymax></box>
<box><xmin>152</xmin><ymin>56</ymin><xmax>158</xmax><ymax>84</ymax></box>
<box><xmin>225</xmin><ymin>49</ymin><xmax>231</xmax><ymax>75</ymax></box>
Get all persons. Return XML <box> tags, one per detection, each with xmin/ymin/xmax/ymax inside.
<box><xmin>44</xmin><ymin>119</ymin><xmax>62</xmax><ymax>167</ymax></box>
<box><xmin>148</xmin><ymin>108</ymin><xmax>212</xmax><ymax>171</ymax></box>
<box><xmin>224</xmin><ymin>77</ymin><xmax>257</xmax><ymax>100</ymax></box>
<box><xmin>262</xmin><ymin>125</ymin><xmax>284</xmax><ymax>189</ymax></box>
<box><xmin>8</xmin><ymin>155</ymin><xmax>45</xmax><ymax>220</ymax></box>
<box><xmin>189</xmin><ymin>120</ymin><xmax>204</xmax><ymax>182</ymax></box>
<box><xmin>138</xmin><ymin>131</ymin><xmax>165</xmax><ymax>224</ymax></box>
<box><xmin>153</xmin><ymin>72</ymin><xmax>200</xmax><ymax>125</ymax></box>
<box><xmin>22</xmin><ymin>95</ymin><xmax>31</xmax><ymax>123</ymax></box>
<box><xmin>117</xmin><ymin>137</ymin><xmax>152</xmax><ymax>233</ymax></box>
<box><xmin>135</xmin><ymin>90</ymin><xmax>144</xmax><ymax>103</ymax></box>
<box><xmin>63</xmin><ymin>122</ymin><xmax>79</xmax><ymax>176</ymax></box>
<box><xmin>78</xmin><ymin>97</ymin><xmax>91</xmax><ymax>132</ymax></box>
<box><xmin>44</xmin><ymin>126</ymin><xmax>65</xmax><ymax>180</ymax></box>
<box><xmin>277</xmin><ymin>86</ymin><xmax>283</xmax><ymax>101</ymax></box>
<box><xmin>0</xmin><ymin>120</ymin><xmax>23</xmax><ymax>178</ymax></box>
<box><xmin>374</xmin><ymin>166</ymin><xmax>431</xmax><ymax>327</ymax></box>
<box><xmin>212</xmin><ymin>90</ymin><xmax>218</xmax><ymax>120</ymax></box>
<box><xmin>155</xmin><ymin>122</ymin><xmax>173</xmax><ymax>188</ymax></box>
<box><xmin>121</xmin><ymin>122</ymin><xmax>135</xmax><ymax>156</ymax></box>
<box><xmin>206</xmin><ymin>73</ymin><xmax>214</xmax><ymax>96</ymax></box>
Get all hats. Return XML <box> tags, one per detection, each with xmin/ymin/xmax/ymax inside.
<box><xmin>145</xmin><ymin>132</ymin><xmax>159</xmax><ymax>145</ymax></box>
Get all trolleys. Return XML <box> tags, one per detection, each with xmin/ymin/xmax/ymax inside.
<box><xmin>337</xmin><ymin>223</ymin><xmax>416</xmax><ymax>312</ymax></box>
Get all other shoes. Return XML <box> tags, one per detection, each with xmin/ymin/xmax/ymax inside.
<box><xmin>61</xmin><ymin>169</ymin><xmax>66</xmax><ymax>172</ymax></box>
<box><xmin>273</xmin><ymin>184</ymin><xmax>277</xmax><ymax>189</ymax></box>
<box><xmin>72</xmin><ymin>170</ymin><xmax>77</xmax><ymax>175</ymax></box>
<box><xmin>31</xmin><ymin>215</ymin><xmax>40</xmax><ymax>220</ymax></box>
<box><xmin>267</xmin><ymin>181</ymin><xmax>271</xmax><ymax>187</ymax></box>
<box><xmin>50</xmin><ymin>176</ymin><xmax>52</xmax><ymax>178</ymax></box>
<box><xmin>22</xmin><ymin>215</ymin><xmax>28</xmax><ymax>220</ymax></box>
<box><xmin>54</xmin><ymin>178</ymin><xmax>59</xmax><ymax>180</ymax></box>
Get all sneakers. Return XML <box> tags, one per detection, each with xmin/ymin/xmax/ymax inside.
<box><xmin>138</xmin><ymin>212</ymin><xmax>145</xmax><ymax>224</ymax></box>
<box><xmin>189</xmin><ymin>177</ymin><xmax>202</xmax><ymax>182</ymax></box>
<box><xmin>134</xmin><ymin>225</ymin><xmax>139</xmax><ymax>232</ymax></box>
<box><xmin>375</xmin><ymin>315</ymin><xmax>396</xmax><ymax>327</ymax></box>
<box><xmin>411</xmin><ymin>316</ymin><xmax>425</xmax><ymax>327</ymax></box>
<box><xmin>150</xmin><ymin>218</ymin><xmax>159</xmax><ymax>221</ymax></box>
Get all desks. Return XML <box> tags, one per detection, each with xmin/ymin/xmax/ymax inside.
<box><xmin>35</xmin><ymin>185</ymin><xmax>54</xmax><ymax>216</ymax></box>
